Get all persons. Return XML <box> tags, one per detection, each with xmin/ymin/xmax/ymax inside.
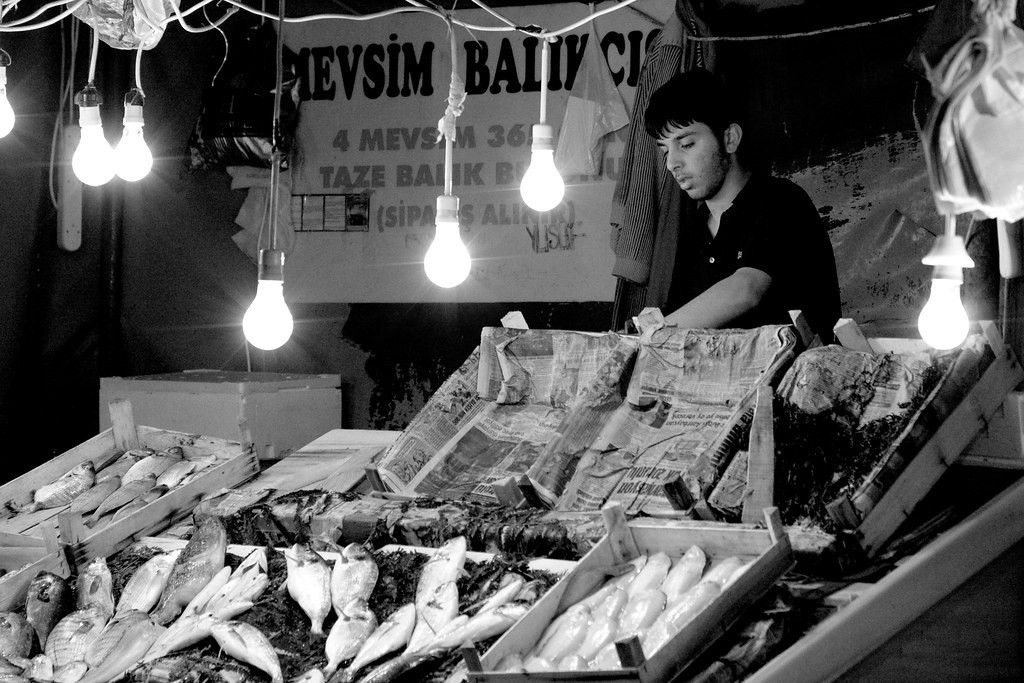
<box><xmin>643</xmin><ymin>70</ymin><xmax>842</xmax><ymax>359</ymax></box>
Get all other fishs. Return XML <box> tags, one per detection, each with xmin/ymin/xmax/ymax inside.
<box><xmin>0</xmin><ymin>440</ymin><xmax>764</xmax><ymax>683</ymax></box>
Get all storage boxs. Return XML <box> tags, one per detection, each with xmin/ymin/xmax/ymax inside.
<box><xmin>520</xmin><ymin>305</ymin><xmax>823</xmax><ymax>518</ymax></box>
<box><xmin>461</xmin><ymin>503</ymin><xmax>792</xmax><ymax>683</ymax></box>
<box><xmin>0</xmin><ymin>523</ymin><xmax>70</xmax><ymax>611</ymax></box>
<box><xmin>100</xmin><ymin>370</ymin><xmax>342</xmax><ymax>461</ymax></box>
<box><xmin>659</xmin><ymin>313</ymin><xmax>1012</xmax><ymax>554</ymax></box>
<box><xmin>0</xmin><ymin>400</ymin><xmax>260</xmax><ymax>573</ymax></box>
<box><xmin>355</xmin><ymin>321</ymin><xmax>640</xmax><ymax>506</ymax></box>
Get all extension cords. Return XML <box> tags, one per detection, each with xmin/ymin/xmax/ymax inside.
<box><xmin>57</xmin><ymin>123</ymin><xmax>82</xmax><ymax>252</ymax></box>
